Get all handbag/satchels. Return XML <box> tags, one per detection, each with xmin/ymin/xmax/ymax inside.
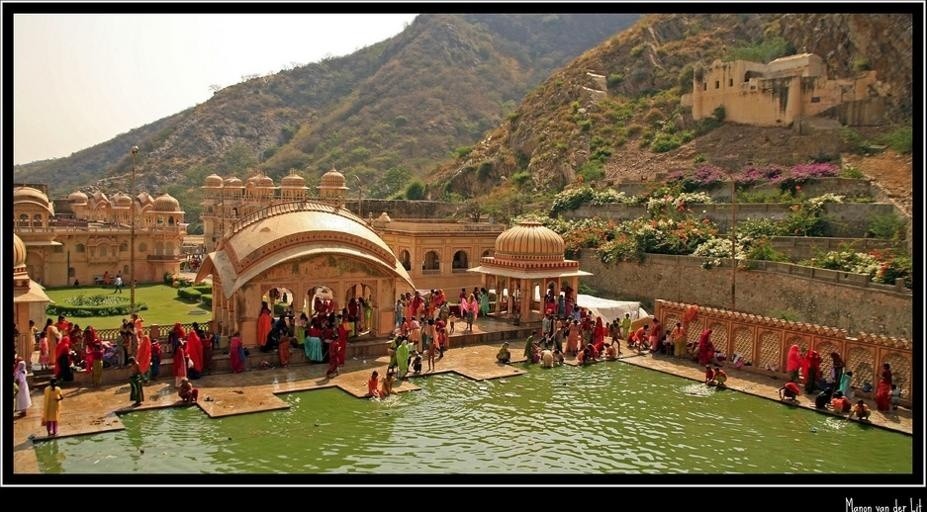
<box><xmin>387</xmin><ymin>347</ymin><xmax>395</xmax><ymax>356</ymax></box>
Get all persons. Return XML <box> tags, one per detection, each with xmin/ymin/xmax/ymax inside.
<box><xmin>13</xmin><ymin>274</ymin><xmax>903</xmax><ymax>441</ymax></box>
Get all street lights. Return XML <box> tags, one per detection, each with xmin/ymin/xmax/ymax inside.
<box><xmin>715</xmin><ymin>171</ymin><xmax>737</xmax><ymax>311</ymax></box>
<box><xmin>127</xmin><ymin>145</ymin><xmax>139</xmax><ymax>317</ymax></box>
<box><xmin>500</xmin><ymin>176</ymin><xmax>511</xmax><ymax>223</ymax></box>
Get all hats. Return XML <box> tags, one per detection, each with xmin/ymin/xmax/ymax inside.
<box><xmin>573</xmin><ymin>320</ymin><xmax>579</xmax><ymax>323</ymax></box>
